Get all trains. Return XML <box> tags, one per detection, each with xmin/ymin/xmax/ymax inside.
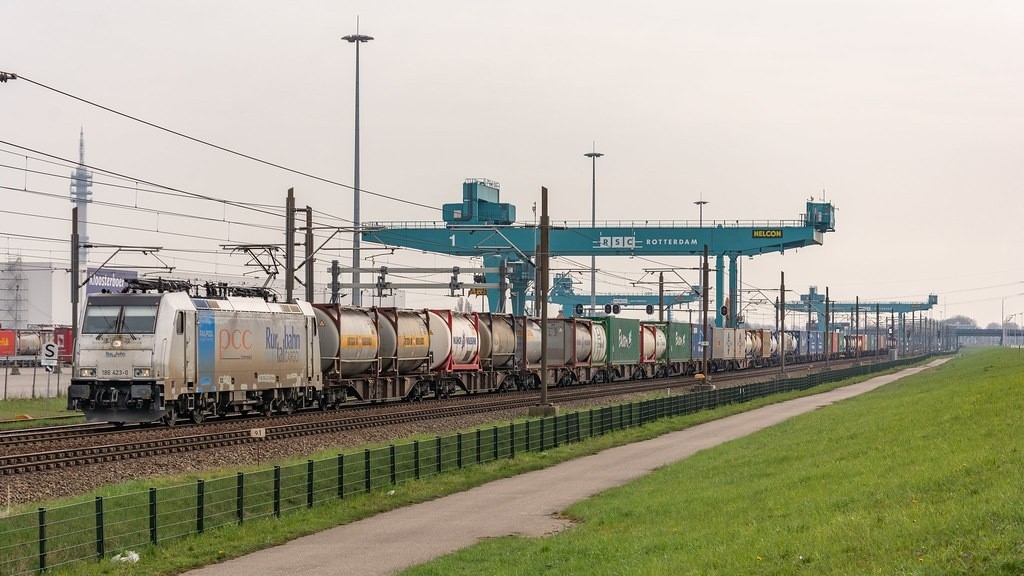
<box><xmin>0</xmin><ymin>324</ymin><xmax>76</xmax><ymax>369</ymax></box>
<box><xmin>63</xmin><ymin>272</ymin><xmax>912</xmax><ymax>428</ymax></box>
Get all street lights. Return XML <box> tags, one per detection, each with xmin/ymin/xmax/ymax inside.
<box><xmin>1000</xmin><ymin>292</ymin><xmax>1024</xmax><ymax>347</ymax></box>
<box><xmin>583</xmin><ymin>140</ymin><xmax>605</xmax><ymax>316</ymax></box>
<box><xmin>341</xmin><ymin>15</ymin><xmax>375</xmax><ymax>307</ymax></box>
<box><xmin>695</xmin><ymin>192</ymin><xmax>711</xmax><ymax>325</ymax></box>
